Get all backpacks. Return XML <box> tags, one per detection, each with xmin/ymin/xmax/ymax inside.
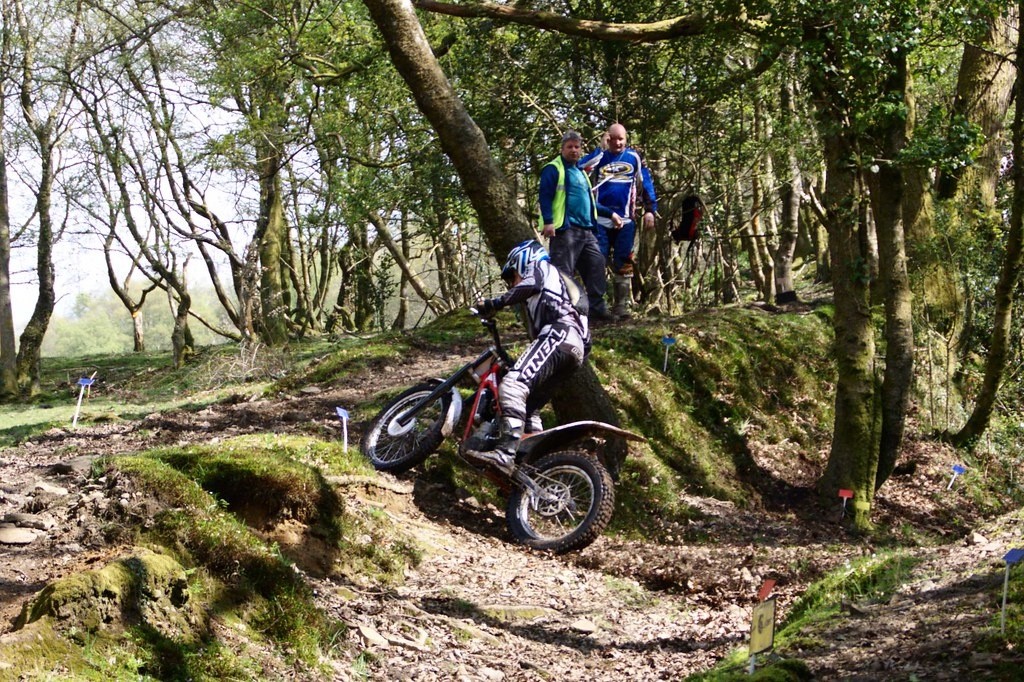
<box><xmin>670</xmin><ymin>195</ymin><xmax>704</xmax><ymax>242</ymax></box>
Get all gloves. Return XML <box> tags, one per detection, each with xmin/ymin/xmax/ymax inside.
<box><xmin>477</xmin><ymin>299</ymin><xmax>499</xmax><ymax>319</ymax></box>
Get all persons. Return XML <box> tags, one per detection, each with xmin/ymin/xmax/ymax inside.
<box><xmin>466</xmin><ymin>238</ymin><xmax>592</xmax><ymax>477</ymax></box>
<box><xmin>577</xmin><ymin>123</ymin><xmax>658</xmax><ymax>320</ymax></box>
<box><xmin>538</xmin><ymin>130</ymin><xmax>625</xmax><ymax>324</ymax></box>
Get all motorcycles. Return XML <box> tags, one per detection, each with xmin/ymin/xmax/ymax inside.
<box><xmin>360</xmin><ymin>301</ymin><xmax>648</xmax><ymax>554</ymax></box>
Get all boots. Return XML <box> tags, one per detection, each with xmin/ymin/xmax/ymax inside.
<box><xmin>466</xmin><ymin>433</ymin><xmax>520</xmax><ymax>479</ymax></box>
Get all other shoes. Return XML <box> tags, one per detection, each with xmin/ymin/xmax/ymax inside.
<box><xmin>612</xmin><ymin>302</ymin><xmax>630</xmax><ymax>320</ymax></box>
<box><xmin>589</xmin><ymin>312</ymin><xmax>614</xmax><ymax>323</ymax></box>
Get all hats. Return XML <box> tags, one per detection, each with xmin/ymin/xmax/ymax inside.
<box><xmin>562</xmin><ymin>130</ymin><xmax>582</xmax><ymax>141</ymax></box>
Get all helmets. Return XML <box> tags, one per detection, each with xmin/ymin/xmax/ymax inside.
<box><xmin>501</xmin><ymin>240</ymin><xmax>550</xmax><ymax>279</ymax></box>
